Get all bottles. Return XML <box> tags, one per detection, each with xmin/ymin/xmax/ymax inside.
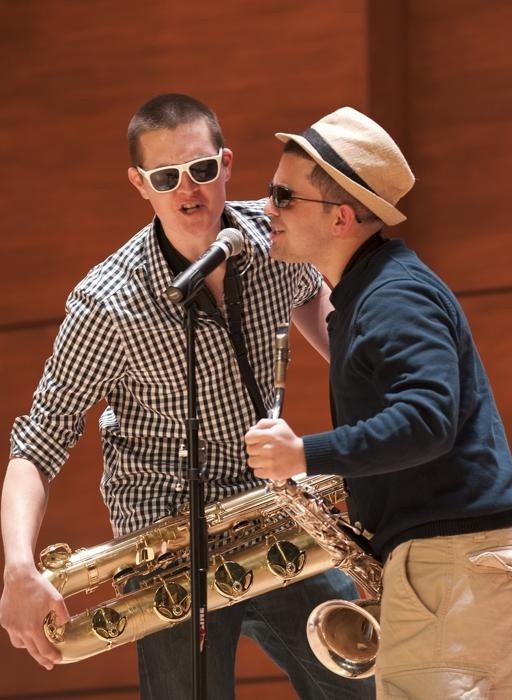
<box><xmin>273</xmin><ymin>106</ymin><xmax>417</xmax><ymax>227</ymax></box>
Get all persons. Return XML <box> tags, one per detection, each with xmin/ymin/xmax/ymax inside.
<box><xmin>244</xmin><ymin>106</ymin><xmax>512</xmax><ymax>700</ymax></box>
<box><xmin>0</xmin><ymin>93</ymin><xmax>379</xmax><ymax>700</ymax></box>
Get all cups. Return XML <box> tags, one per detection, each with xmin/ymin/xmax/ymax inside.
<box><xmin>267</xmin><ymin>181</ymin><xmax>362</xmax><ymax>224</ymax></box>
<box><xmin>134</xmin><ymin>147</ymin><xmax>225</xmax><ymax>194</ymax></box>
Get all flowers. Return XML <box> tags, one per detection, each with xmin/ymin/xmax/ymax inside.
<box><xmin>166</xmin><ymin>227</ymin><xmax>245</xmax><ymax>302</ymax></box>
